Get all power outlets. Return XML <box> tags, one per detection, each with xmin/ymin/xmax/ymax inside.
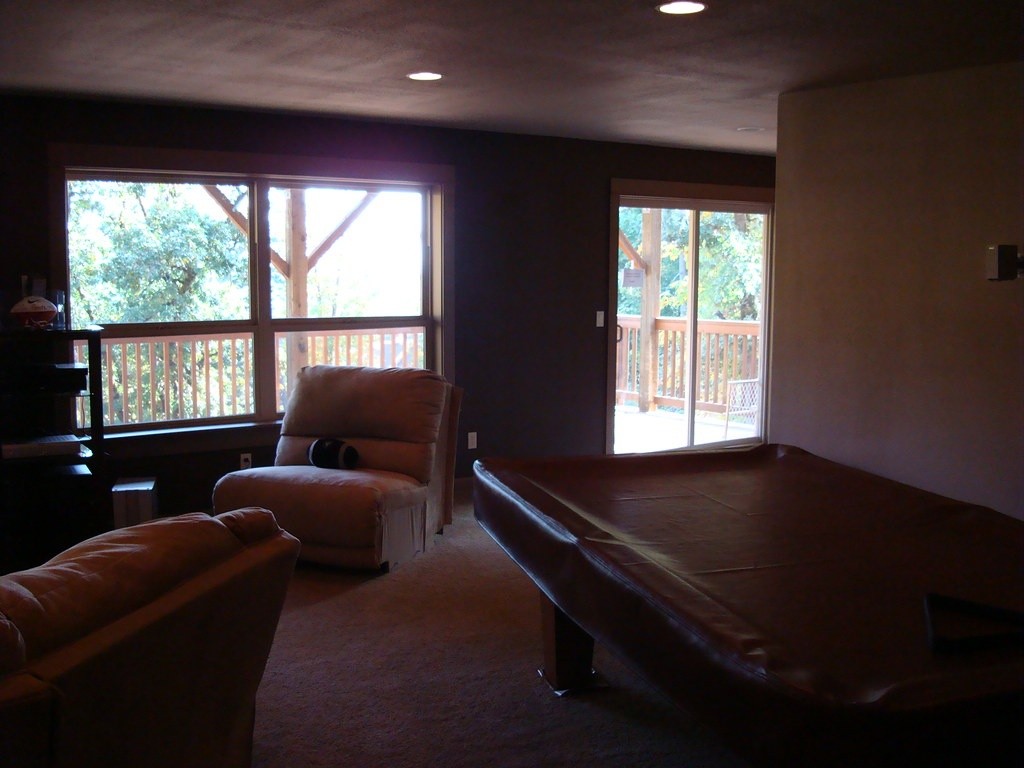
<box><xmin>240</xmin><ymin>453</ymin><xmax>251</xmax><ymax>469</ymax></box>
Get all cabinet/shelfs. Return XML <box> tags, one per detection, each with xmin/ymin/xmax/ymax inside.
<box><xmin>0</xmin><ymin>263</ymin><xmax>108</xmax><ymax>578</ymax></box>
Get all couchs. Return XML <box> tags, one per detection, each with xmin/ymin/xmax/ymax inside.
<box><xmin>0</xmin><ymin>506</ymin><xmax>299</xmax><ymax>768</ymax></box>
<box><xmin>211</xmin><ymin>368</ymin><xmax>465</xmax><ymax>579</ymax></box>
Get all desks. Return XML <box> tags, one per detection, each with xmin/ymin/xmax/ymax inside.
<box><xmin>469</xmin><ymin>444</ymin><xmax>1024</xmax><ymax>768</ymax></box>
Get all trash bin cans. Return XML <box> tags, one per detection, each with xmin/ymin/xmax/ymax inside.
<box><xmin>112</xmin><ymin>476</ymin><xmax>158</xmax><ymax>528</ymax></box>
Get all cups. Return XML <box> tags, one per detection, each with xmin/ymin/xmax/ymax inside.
<box><xmin>51</xmin><ymin>288</ymin><xmax>66</xmax><ymax>330</ymax></box>
